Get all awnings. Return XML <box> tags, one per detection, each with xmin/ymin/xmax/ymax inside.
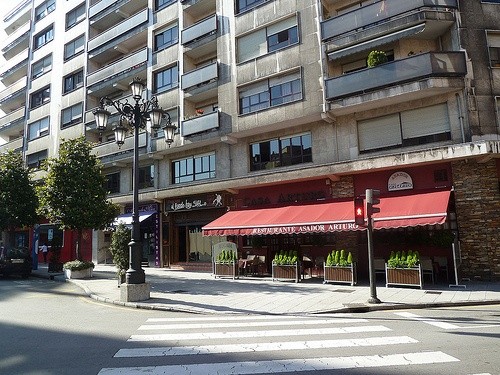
<box><xmin>109</xmin><ymin>210</ymin><xmax>157</xmax><ymax>226</ymax></box>
<box><xmin>202</xmin><ymin>190</ymin><xmax>452</xmax><ymax>237</ymax></box>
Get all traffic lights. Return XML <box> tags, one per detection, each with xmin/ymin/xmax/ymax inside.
<box><xmin>354</xmin><ymin>198</ymin><xmax>365</xmax><ymax>225</ymax></box>
<box><xmin>366</xmin><ymin>188</ymin><xmax>380</xmax><ymax>215</ymax></box>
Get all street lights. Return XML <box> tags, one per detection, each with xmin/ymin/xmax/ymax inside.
<box><xmin>92</xmin><ymin>74</ymin><xmax>178</xmax><ymax>284</ymax></box>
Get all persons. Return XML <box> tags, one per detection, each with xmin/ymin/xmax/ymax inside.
<box><xmin>40</xmin><ymin>242</ymin><xmax>47</xmax><ymax>263</ymax></box>
